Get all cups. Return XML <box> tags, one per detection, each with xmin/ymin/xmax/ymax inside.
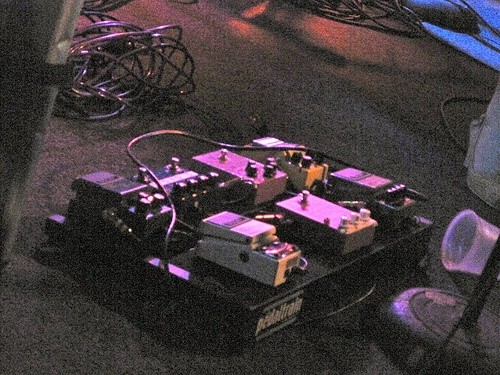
<box><xmin>439</xmin><ymin>210</ymin><xmax>500</xmax><ymax>282</ymax></box>
